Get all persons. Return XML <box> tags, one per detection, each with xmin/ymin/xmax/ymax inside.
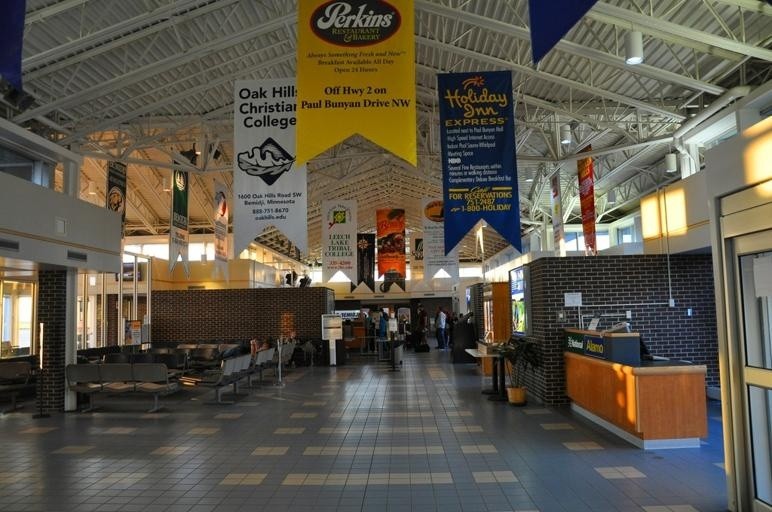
<box><xmin>366</xmin><ymin>306</ymin><xmax>467</xmax><ymax>353</ymax></box>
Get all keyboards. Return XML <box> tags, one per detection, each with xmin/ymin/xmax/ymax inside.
<box><xmin>647</xmin><ymin>356</ymin><xmax>669</xmax><ymax>360</ymax></box>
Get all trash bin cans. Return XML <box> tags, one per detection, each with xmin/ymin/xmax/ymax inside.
<box><xmin>392</xmin><ymin>341</ymin><xmax>405</xmax><ymax>368</ymax></box>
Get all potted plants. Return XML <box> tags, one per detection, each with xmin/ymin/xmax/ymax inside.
<box><xmin>492</xmin><ymin>334</ymin><xmax>544</xmax><ymax>407</ymax></box>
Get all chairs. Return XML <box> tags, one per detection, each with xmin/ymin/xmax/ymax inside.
<box><xmin>59</xmin><ymin>338</ymin><xmax>320</xmax><ymax>420</ymax></box>
<box><xmin>1</xmin><ymin>361</ymin><xmax>32</xmax><ymax>415</ymax></box>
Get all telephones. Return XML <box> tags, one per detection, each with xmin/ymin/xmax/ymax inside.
<box><xmin>607</xmin><ymin>322</ymin><xmax>630</xmax><ymax>333</ymax></box>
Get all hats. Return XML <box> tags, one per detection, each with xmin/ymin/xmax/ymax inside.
<box><xmin>416</xmin><ymin>303</ymin><xmax>424</xmax><ymax>307</ymax></box>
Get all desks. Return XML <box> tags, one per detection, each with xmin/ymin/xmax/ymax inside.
<box><xmin>375</xmin><ymin>337</ymin><xmax>392</xmax><ymax>362</ymax></box>
<box><xmin>356</xmin><ymin>336</ymin><xmax>381</xmax><ymax>356</ymax></box>
<box><xmin>464</xmin><ymin>348</ymin><xmax>510</xmax><ymax>402</ymax></box>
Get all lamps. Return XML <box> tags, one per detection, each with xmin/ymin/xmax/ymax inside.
<box><xmin>623</xmin><ymin>20</ymin><xmax>645</xmax><ymax>65</ymax></box>
<box><xmin>88</xmin><ymin>180</ymin><xmax>97</xmax><ymax>195</ymax></box>
<box><xmin>161</xmin><ymin>177</ymin><xmax>171</xmax><ymax>191</ymax></box>
<box><xmin>664</xmin><ymin>143</ymin><xmax>679</xmax><ymax>173</ymax></box>
<box><xmin>560</xmin><ymin>124</ymin><xmax>572</xmax><ymax>144</ymax></box>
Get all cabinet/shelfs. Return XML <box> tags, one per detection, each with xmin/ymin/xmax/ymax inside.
<box><xmin>482</xmin><ymin>281</ymin><xmax>511</xmax><ymax>346</ymax></box>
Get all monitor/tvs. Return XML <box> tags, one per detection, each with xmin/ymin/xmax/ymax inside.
<box><xmin>116</xmin><ymin>263</ymin><xmax>141</xmax><ymax>281</ymax></box>
<box><xmin>639</xmin><ymin>336</ymin><xmax>650</xmax><ymax>360</ymax></box>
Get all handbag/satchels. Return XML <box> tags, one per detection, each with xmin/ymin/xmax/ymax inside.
<box><xmin>415</xmin><ymin>344</ymin><xmax>430</xmax><ymax>352</ymax></box>
